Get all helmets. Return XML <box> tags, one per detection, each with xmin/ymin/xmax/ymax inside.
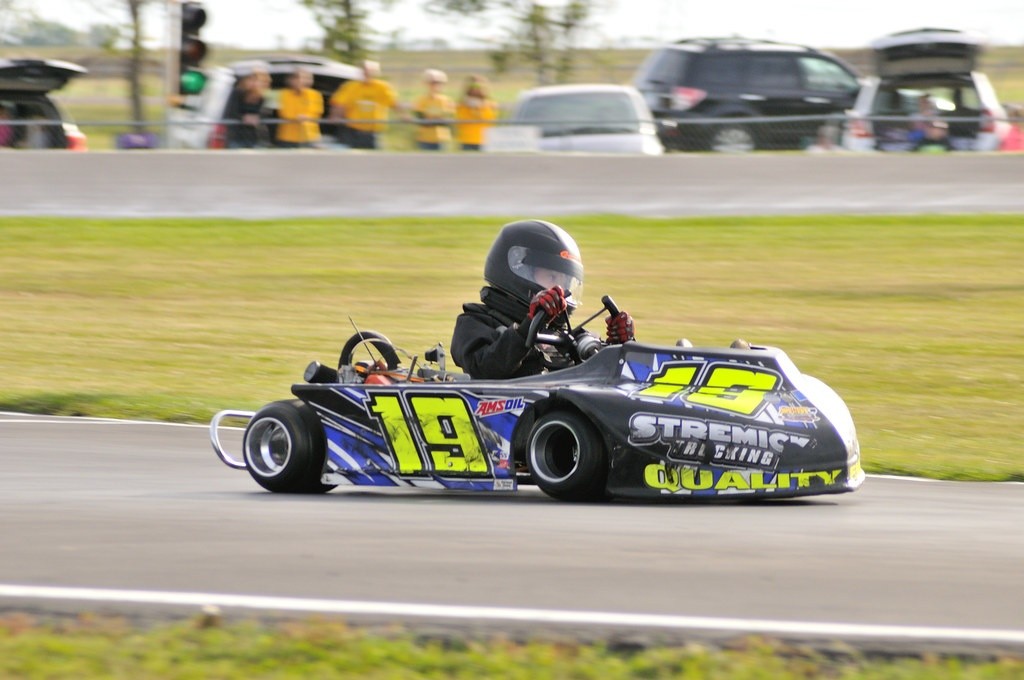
<box><xmin>483</xmin><ymin>220</ymin><xmax>584</xmax><ymax>329</ymax></box>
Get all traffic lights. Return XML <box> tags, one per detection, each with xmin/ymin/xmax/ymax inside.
<box><xmin>165</xmin><ymin>0</ymin><xmax>208</xmax><ymax>111</ymax></box>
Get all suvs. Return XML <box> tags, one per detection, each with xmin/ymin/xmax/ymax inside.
<box><xmin>635</xmin><ymin>37</ymin><xmax>963</xmax><ymax>152</ymax></box>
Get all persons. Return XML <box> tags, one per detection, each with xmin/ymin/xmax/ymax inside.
<box><xmin>412</xmin><ymin>69</ymin><xmax>498</xmax><ymax>151</ymax></box>
<box><xmin>221</xmin><ymin>62</ymin><xmax>323</xmax><ymax>148</ymax></box>
<box><xmin>806</xmin><ymin>95</ymin><xmax>1024</xmax><ymax>151</ymax></box>
<box><xmin>451</xmin><ymin>220</ymin><xmax>634</xmax><ymax>379</ymax></box>
<box><xmin>329</xmin><ymin>59</ymin><xmax>412</xmax><ymax>150</ymax></box>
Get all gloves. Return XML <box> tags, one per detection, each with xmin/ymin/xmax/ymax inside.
<box><xmin>605</xmin><ymin>311</ymin><xmax>636</xmax><ymax>345</ymax></box>
<box><xmin>528</xmin><ymin>284</ymin><xmax>567</xmax><ymax>320</ymax></box>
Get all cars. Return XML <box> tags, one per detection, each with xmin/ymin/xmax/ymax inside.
<box><xmin>486</xmin><ymin>83</ymin><xmax>667</xmax><ymax>156</ymax></box>
<box><xmin>167</xmin><ymin>55</ymin><xmax>379</xmax><ymax>156</ymax></box>
<box><xmin>0</xmin><ymin>56</ymin><xmax>89</xmax><ymax>153</ymax></box>
<box><xmin>835</xmin><ymin>27</ymin><xmax>1014</xmax><ymax>152</ymax></box>
